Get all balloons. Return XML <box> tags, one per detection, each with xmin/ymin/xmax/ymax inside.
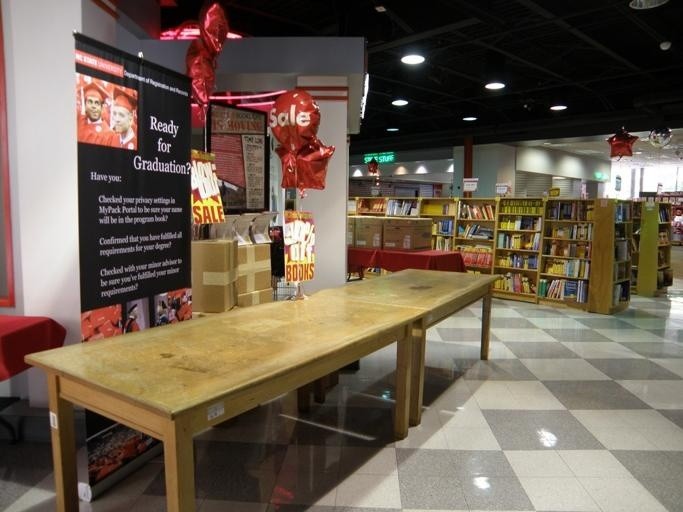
<box><xmin>648</xmin><ymin>128</ymin><xmax>673</xmax><ymax>149</ymax></box>
<box><xmin>367</xmin><ymin>157</ymin><xmax>378</xmax><ymax>174</ymax></box>
<box><xmin>274</xmin><ymin>137</ymin><xmax>335</xmax><ymax>189</ymax></box>
<box><xmin>187</xmin><ymin>39</ymin><xmax>215</xmax><ymax>108</ymax></box>
<box><xmin>269</xmin><ymin>89</ymin><xmax>320</xmax><ymax>155</ymax></box>
<box><xmin>605</xmin><ymin>126</ymin><xmax>638</xmax><ymax>156</ymax></box>
<box><xmin>199</xmin><ymin>4</ymin><xmax>227</xmax><ymax>51</ymax></box>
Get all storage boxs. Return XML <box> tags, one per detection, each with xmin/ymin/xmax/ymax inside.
<box><xmin>183</xmin><ymin>234</ymin><xmax>278</xmax><ymax>322</ymax></box>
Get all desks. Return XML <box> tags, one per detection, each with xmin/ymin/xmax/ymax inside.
<box><xmin>0</xmin><ymin>311</ymin><xmax>68</xmax><ymax>444</ymax></box>
<box><xmin>19</xmin><ymin>298</ymin><xmax>430</xmax><ymax>510</ymax></box>
<box><xmin>290</xmin><ymin>264</ymin><xmax>504</xmax><ymax>428</ymax></box>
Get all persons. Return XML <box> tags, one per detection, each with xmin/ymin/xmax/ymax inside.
<box><xmin>99</xmin><ymin>104</ymin><xmax>137</xmax><ymax>150</ymax></box>
<box><xmin>671</xmin><ymin>206</ymin><xmax>682</xmax><ymax>228</ymax></box>
<box><xmin>81</xmin><ymin>288</ymin><xmax>192</xmax><ymax>336</ymax></box>
<box><xmin>77</xmin><ymin>90</ymin><xmax>116</xmax><ymax>146</ymax></box>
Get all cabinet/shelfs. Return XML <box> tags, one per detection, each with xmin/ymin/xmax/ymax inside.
<box><xmin>346</xmin><ymin>193</ymin><xmax>682</xmax><ymax>318</ymax></box>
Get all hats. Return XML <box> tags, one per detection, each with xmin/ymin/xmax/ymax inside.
<box><xmin>127</xmin><ymin>304</ymin><xmax>137</xmax><ymax>315</ymax></box>
<box><xmin>156</xmin><ymin>288</ymin><xmax>192</xmax><ymax>326</ymax></box>
<box><xmin>81</xmin><ymin>304</ymin><xmax>122</xmax><ymax>342</ymax></box>
<box><xmin>78</xmin><ymin>82</ymin><xmax>110</xmax><ymax>102</ymax></box>
<box><xmin>112</xmin><ymin>87</ymin><xmax>137</xmax><ymax>114</ymax></box>
